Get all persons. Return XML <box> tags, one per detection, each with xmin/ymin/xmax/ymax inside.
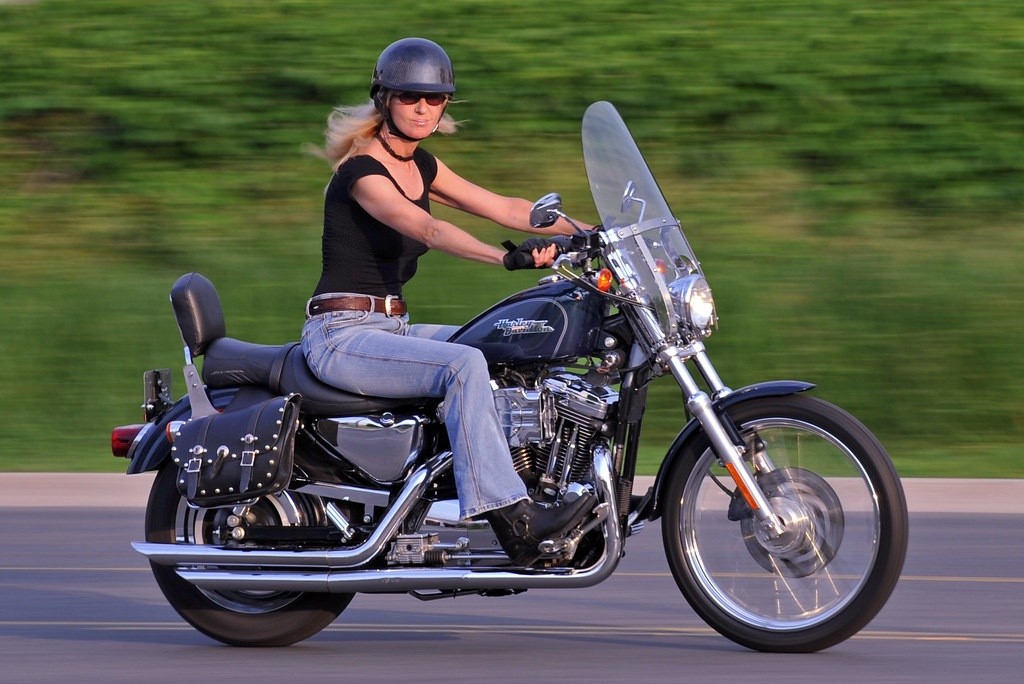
<box><xmin>301</xmin><ymin>38</ymin><xmax>607</xmax><ymax>572</ymax></box>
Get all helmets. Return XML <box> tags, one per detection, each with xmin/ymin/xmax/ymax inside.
<box><xmin>369</xmin><ymin>37</ymin><xmax>456</xmax><ymax>99</ymax></box>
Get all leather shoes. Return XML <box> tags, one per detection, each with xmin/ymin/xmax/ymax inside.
<box><xmin>485</xmin><ymin>491</ymin><xmax>599</xmax><ymax>570</ymax></box>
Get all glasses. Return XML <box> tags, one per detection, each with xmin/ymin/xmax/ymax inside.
<box><xmin>390</xmin><ymin>91</ymin><xmax>448</xmax><ymax>106</ymax></box>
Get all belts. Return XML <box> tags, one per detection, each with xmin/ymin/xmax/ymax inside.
<box><xmin>304</xmin><ymin>297</ymin><xmax>408</xmax><ymax>320</ymax></box>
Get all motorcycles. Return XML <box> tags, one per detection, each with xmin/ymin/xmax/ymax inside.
<box><xmin>108</xmin><ymin>96</ymin><xmax>912</xmax><ymax>654</ymax></box>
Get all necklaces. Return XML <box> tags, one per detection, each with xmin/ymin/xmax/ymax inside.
<box><xmin>372</xmin><ymin>130</ymin><xmax>416</xmax><ymax>162</ymax></box>
